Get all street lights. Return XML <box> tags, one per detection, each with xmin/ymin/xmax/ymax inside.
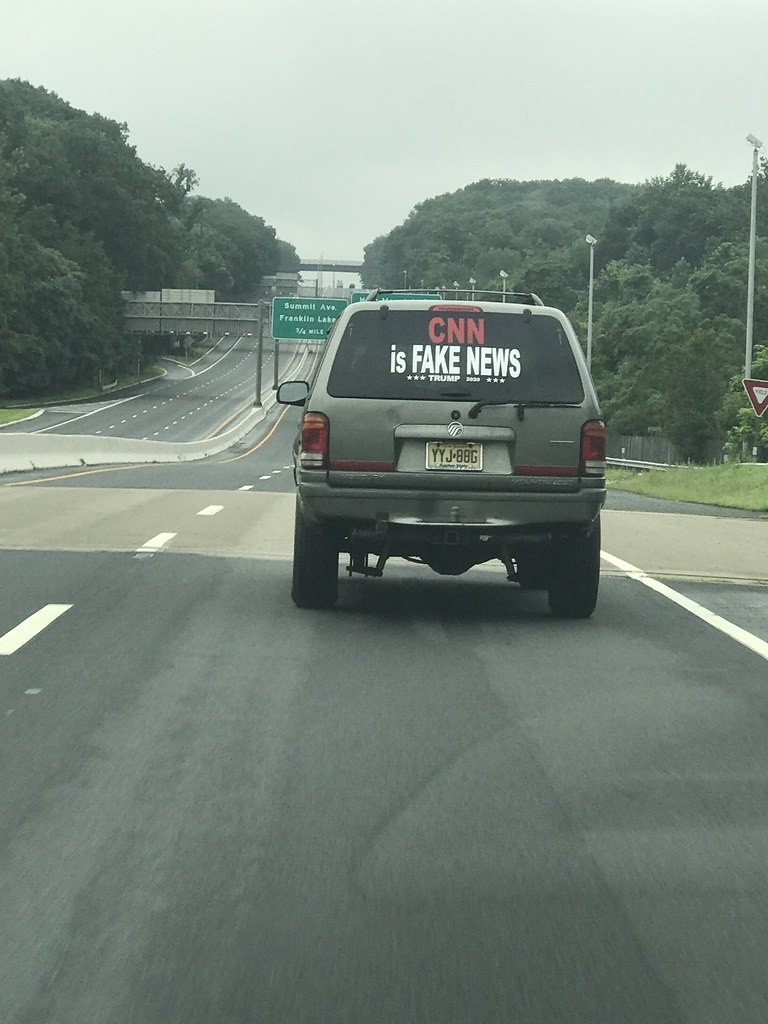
<box><xmin>584</xmin><ymin>233</ymin><xmax>598</xmax><ymax>370</ymax></box>
<box><xmin>498</xmin><ymin>268</ymin><xmax>511</xmax><ymax>301</ymax></box>
<box><xmin>742</xmin><ymin>132</ymin><xmax>764</xmax><ymax>464</ymax></box>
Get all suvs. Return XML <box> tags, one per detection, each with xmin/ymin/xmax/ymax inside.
<box><xmin>276</xmin><ymin>287</ymin><xmax>607</xmax><ymax>616</ymax></box>
